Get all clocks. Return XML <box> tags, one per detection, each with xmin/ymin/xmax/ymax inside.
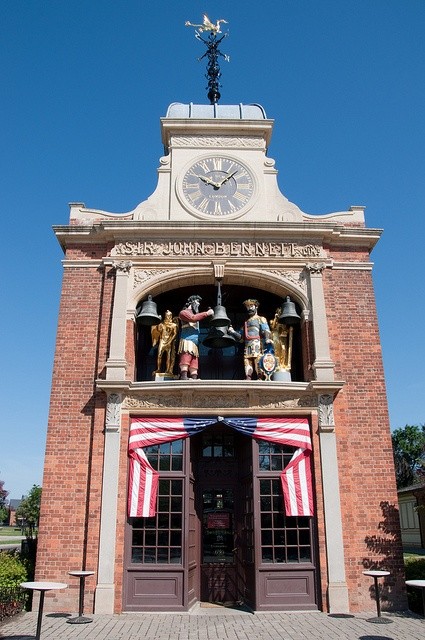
<box><xmin>175</xmin><ymin>151</ymin><xmax>260</xmax><ymax>220</ymax></box>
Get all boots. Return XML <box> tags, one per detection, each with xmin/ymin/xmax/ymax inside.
<box><xmin>181</xmin><ymin>370</ymin><xmax>190</xmax><ymax>380</ymax></box>
<box><xmin>244</xmin><ymin>375</ymin><xmax>251</xmax><ymax>380</ymax></box>
<box><xmin>191</xmin><ymin>374</ymin><xmax>201</xmax><ymax>379</ymax></box>
<box><xmin>257</xmin><ymin>373</ymin><xmax>262</xmax><ymax>381</ymax></box>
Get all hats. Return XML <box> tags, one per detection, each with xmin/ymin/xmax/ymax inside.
<box><xmin>243</xmin><ymin>299</ymin><xmax>260</xmax><ymax>303</ymax></box>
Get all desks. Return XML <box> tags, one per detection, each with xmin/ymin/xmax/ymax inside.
<box><xmin>404</xmin><ymin>578</ymin><xmax>425</xmax><ymax>588</ymax></box>
<box><xmin>19</xmin><ymin>582</ymin><xmax>67</xmax><ymax>640</ymax></box>
<box><xmin>67</xmin><ymin>569</ymin><xmax>95</xmax><ymax>625</ymax></box>
<box><xmin>362</xmin><ymin>570</ymin><xmax>391</xmax><ymax>623</ymax></box>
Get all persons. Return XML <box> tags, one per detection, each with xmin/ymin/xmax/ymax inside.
<box><xmin>177</xmin><ymin>295</ymin><xmax>214</xmax><ymax>381</ymax></box>
<box><xmin>151</xmin><ymin>310</ymin><xmax>181</xmax><ymax>375</ymax></box>
<box><xmin>269</xmin><ymin>307</ymin><xmax>289</xmax><ymax>368</ymax></box>
<box><xmin>228</xmin><ymin>299</ymin><xmax>270</xmax><ymax>381</ymax></box>
